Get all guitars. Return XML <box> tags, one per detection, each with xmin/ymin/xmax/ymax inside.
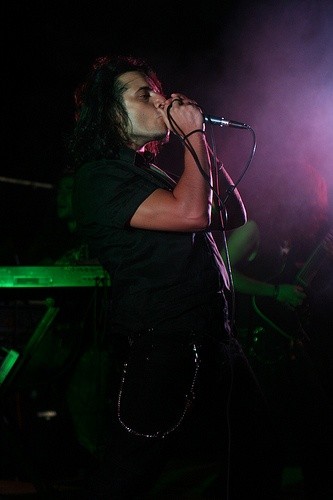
<box><xmin>247</xmin><ymin>228</ymin><xmax>333</xmax><ymax>365</ymax></box>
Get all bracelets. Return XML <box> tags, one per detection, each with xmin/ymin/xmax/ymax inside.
<box><xmin>181</xmin><ymin>130</ymin><xmax>205</xmax><ymax>141</ymax></box>
<box><xmin>213</xmin><ymin>161</ymin><xmax>224</xmax><ymax>175</ymax></box>
<box><xmin>274</xmin><ymin>283</ymin><xmax>279</xmax><ymax>299</ymax></box>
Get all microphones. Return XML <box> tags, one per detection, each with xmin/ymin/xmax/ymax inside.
<box><xmin>204</xmin><ymin>113</ymin><xmax>250</xmax><ymax>129</ymax></box>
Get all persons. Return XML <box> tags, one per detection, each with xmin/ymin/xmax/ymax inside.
<box><xmin>53</xmin><ymin>165</ymin><xmax>118</xmax><ymax>461</ymax></box>
<box><xmin>72</xmin><ymin>54</ymin><xmax>282</xmax><ymax>500</ymax></box>
<box><xmin>217</xmin><ymin>161</ymin><xmax>333</xmax><ymax>405</ymax></box>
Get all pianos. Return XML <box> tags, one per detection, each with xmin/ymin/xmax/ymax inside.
<box><xmin>0</xmin><ymin>262</ymin><xmax>113</xmax><ymax>292</ymax></box>
